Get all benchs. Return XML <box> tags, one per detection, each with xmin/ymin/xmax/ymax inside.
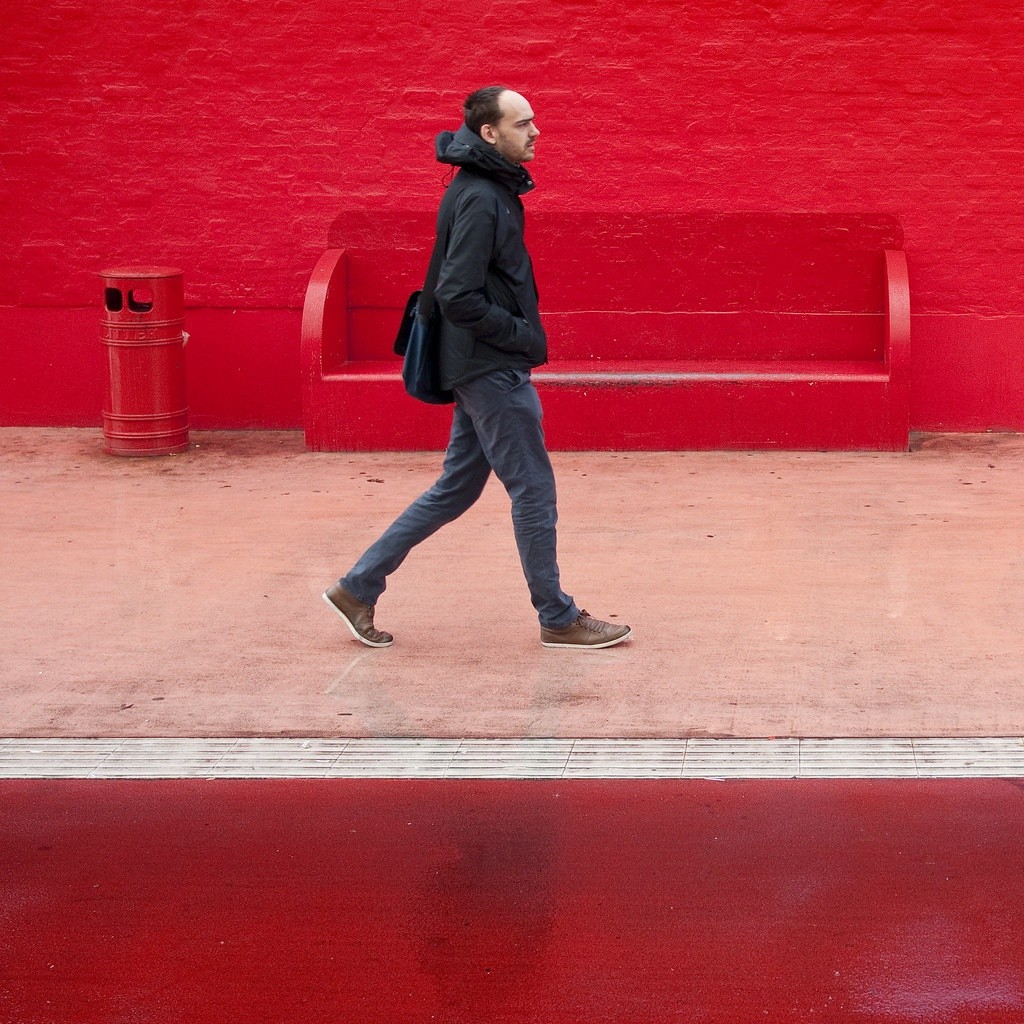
<box><xmin>298</xmin><ymin>208</ymin><xmax>914</xmax><ymax>452</ymax></box>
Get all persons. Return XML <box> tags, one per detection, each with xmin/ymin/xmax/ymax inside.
<box><xmin>322</xmin><ymin>85</ymin><xmax>631</xmax><ymax>648</ymax></box>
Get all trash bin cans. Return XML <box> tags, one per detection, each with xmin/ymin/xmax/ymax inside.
<box><xmin>96</xmin><ymin>264</ymin><xmax>192</xmax><ymax>460</ymax></box>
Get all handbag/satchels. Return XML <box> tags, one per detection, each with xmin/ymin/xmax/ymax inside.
<box><xmin>394</xmin><ymin>289</ymin><xmax>455</xmax><ymax>405</ymax></box>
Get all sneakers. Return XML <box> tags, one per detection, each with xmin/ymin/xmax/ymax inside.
<box><xmin>541</xmin><ymin>609</ymin><xmax>632</xmax><ymax>649</ymax></box>
<box><xmin>322</xmin><ymin>580</ymin><xmax>394</xmax><ymax>647</ymax></box>
